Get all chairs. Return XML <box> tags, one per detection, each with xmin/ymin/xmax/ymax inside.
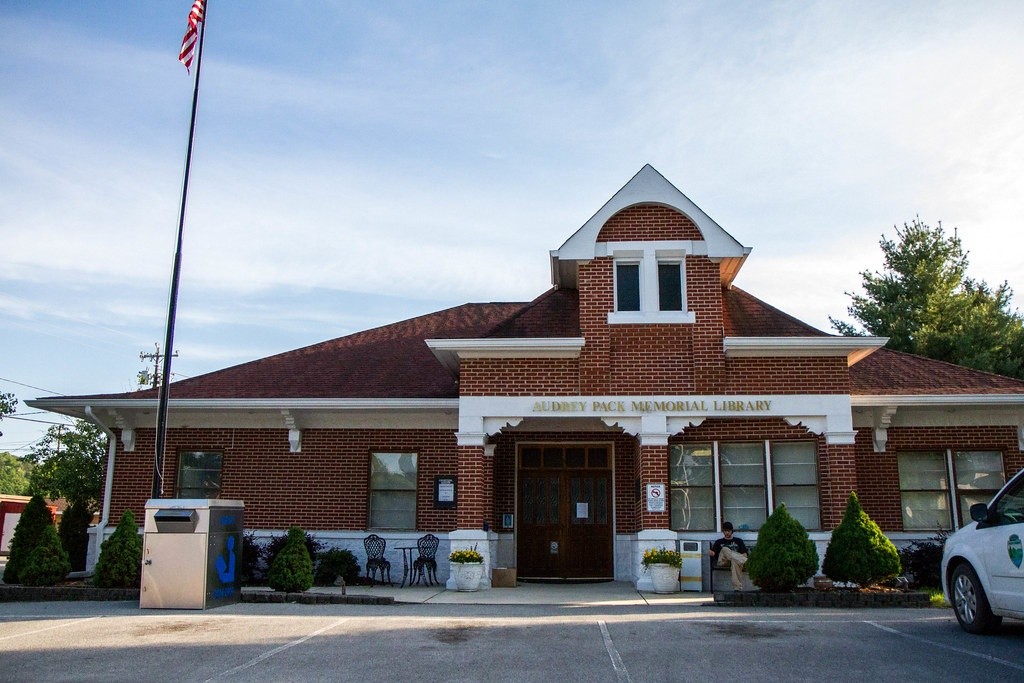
<box><xmin>411</xmin><ymin>534</ymin><xmax>440</xmax><ymax>586</ymax></box>
<box><xmin>363</xmin><ymin>534</ymin><xmax>393</xmax><ymax>588</ymax></box>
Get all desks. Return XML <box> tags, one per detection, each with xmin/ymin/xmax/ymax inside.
<box><xmin>393</xmin><ymin>546</ymin><xmax>431</xmax><ymax>589</ymax></box>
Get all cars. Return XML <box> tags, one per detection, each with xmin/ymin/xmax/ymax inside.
<box><xmin>941</xmin><ymin>468</ymin><xmax>1024</xmax><ymax>635</ymax></box>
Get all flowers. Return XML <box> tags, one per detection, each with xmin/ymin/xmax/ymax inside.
<box><xmin>641</xmin><ymin>547</ymin><xmax>683</xmax><ymax>575</ymax></box>
<box><xmin>447</xmin><ymin>542</ymin><xmax>486</xmax><ymax>564</ymax></box>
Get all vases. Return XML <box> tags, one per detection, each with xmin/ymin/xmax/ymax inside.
<box><xmin>647</xmin><ymin>563</ymin><xmax>680</xmax><ymax>593</ymax></box>
<box><xmin>450</xmin><ymin>561</ymin><xmax>483</xmax><ymax>592</ymax></box>
<box><xmin>813</xmin><ymin>575</ymin><xmax>834</xmax><ymax>590</ymax></box>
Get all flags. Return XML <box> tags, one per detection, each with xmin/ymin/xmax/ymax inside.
<box><xmin>178</xmin><ymin>0</ymin><xmax>205</xmax><ymax>75</ymax></box>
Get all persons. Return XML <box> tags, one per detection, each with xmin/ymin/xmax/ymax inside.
<box><xmin>708</xmin><ymin>522</ymin><xmax>748</xmax><ymax>592</ymax></box>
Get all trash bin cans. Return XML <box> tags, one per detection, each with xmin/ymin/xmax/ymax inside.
<box><xmin>679</xmin><ymin>540</ymin><xmax>703</xmax><ymax>592</ymax></box>
<box><xmin>139</xmin><ymin>498</ymin><xmax>246</xmax><ymax>609</ymax></box>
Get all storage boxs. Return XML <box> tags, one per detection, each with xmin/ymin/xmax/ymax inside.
<box><xmin>492</xmin><ymin>567</ymin><xmax>517</xmax><ymax>588</ymax></box>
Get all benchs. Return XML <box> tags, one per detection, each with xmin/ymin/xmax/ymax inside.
<box><xmin>710</xmin><ymin>541</ymin><xmax>756</xmax><ymax>594</ymax></box>
